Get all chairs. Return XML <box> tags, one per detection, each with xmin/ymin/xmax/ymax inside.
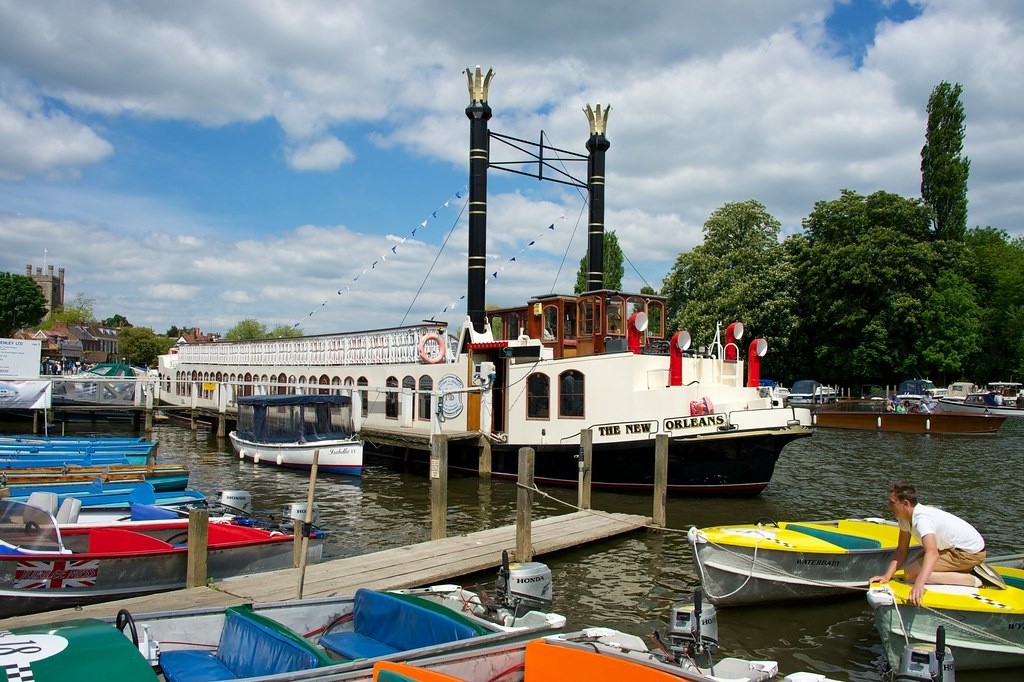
<box><xmin>54</xmin><ymin>497</ymin><xmax>82</xmax><ymax>524</ymax></box>
<box><xmin>21</xmin><ymin>491</ymin><xmax>58</xmax><ymax>525</ymax></box>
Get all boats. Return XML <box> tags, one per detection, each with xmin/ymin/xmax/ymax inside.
<box><xmin>229</xmin><ymin>394</ymin><xmax>363</xmax><ymax>477</ymax></box>
<box><xmin>867</xmin><ymin>554</ymin><xmax>1024</xmax><ymax>675</ymax></box>
<box><xmin>154</xmin><ymin>64</ymin><xmax>813</xmax><ymax>514</ymax></box>
<box><xmin>807</xmin><ymin>409</ymin><xmax>1008</xmax><ymax>438</ymax></box>
<box><xmin>988</xmin><ymin>382</ymin><xmax>1024</xmax><ymax>405</ymax></box>
<box><xmin>934</xmin><ymin>391</ymin><xmax>1024</xmax><ymax>416</ymax></box>
<box><xmin>894</xmin><ymin>378</ymin><xmax>947</xmax><ymax>406</ymax></box>
<box><xmin>687</xmin><ymin>518</ymin><xmax>925</xmax><ymax>606</ymax></box>
<box><xmin>933</xmin><ymin>380</ymin><xmax>979</xmax><ymax>398</ymax></box>
<box><xmin>787</xmin><ymin>381</ymin><xmax>836</xmax><ymax>409</ymax></box>
<box><xmin>759</xmin><ymin>380</ymin><xmax>790</xmax><ymax>408</ymax></box>
<box><xmin>0</xmin><ymin>437</ymin><xmax>327</xmax><ymax>605</ymax></box>
<box><xmin>0</xmin><ymin>550</ymin><xmax>569</xmax><ymax>682</ymax></box>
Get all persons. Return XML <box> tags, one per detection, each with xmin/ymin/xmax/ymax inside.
<box><xmin>868</xmin><ymin>481</ymin><xmax>1007</xmax><ymax>605</ymax></box>
<box><xmin>42</xmin><ymin>358</ymin><xmax>93</xmax><ymax>375</ymax></box>
<box><xmin>917</xmin><ymin>391</ymin><xmax>938</xmax><ymax>413</ymax></box>
<box><xmin>992</xmin><ymin>385</ymin><xmax>1011</xmax><ymax>406</ymax></box>
<box><xmin>1016</xmin><ymin>394</ymin><xmax>1024</xmax><ymax>407</ymax></box>
<box><xmin>885</xmin><ymin>393</ymin><xmax>913</xmax><ymax>413</ymax></box>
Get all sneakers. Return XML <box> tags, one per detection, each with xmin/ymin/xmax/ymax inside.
<box><xmin>971</xmin><ymin>562</ymin><xmax>1007</xmax><ymax>591</ymax></box>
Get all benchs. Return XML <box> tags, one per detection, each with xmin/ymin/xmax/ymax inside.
<box><xmin>785</xmin><ymin>523</ymin><xmax>881</xmax><ymax>549</ymax></box>
<box><xmin>372</xmin><ymin>661</ymin><xmax>469</xmax><ymax>682</ymax></box>
<box><xmin>524</xmin><ymin>639</ymin><xmax>690</xmax><ymax>682</ymax></box>
<box><xmin>320</xmin><ymin>588</ymin><xmax>476</xmax><ymax>662</ymax></box>
<box><xmin>159</xmin><ymin>609</ymin><xmax>319</xmax><ymax>682</ymax></box>
<box><xmin>130</xmin><ymin>503</ymin><xmax>179</xmax><ymax>520</ymax></box>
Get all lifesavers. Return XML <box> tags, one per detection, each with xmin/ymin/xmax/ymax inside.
<box><xmin>418</xmin><ymin>333</ymin><xmax>447</xmax><ymax>364</ymax></box>
